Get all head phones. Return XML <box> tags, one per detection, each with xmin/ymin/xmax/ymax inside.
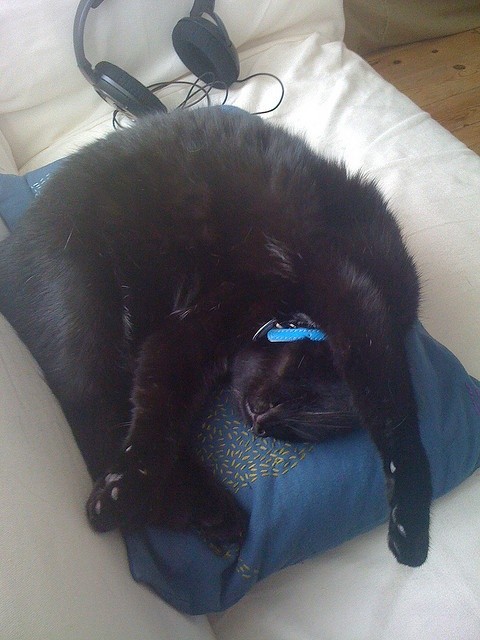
<box><xmin>73</xmin><ymin>0</ymin><xmax>240</xmax><ymax>122</ymax></box>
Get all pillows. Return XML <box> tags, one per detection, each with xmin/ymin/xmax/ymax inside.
<box><xmin>2</xmin><ymin>148</ymin><xmax>478</xmax><ymax>616</ymax></box>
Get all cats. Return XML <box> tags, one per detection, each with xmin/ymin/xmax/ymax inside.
<box><xmin>0</xmin><ymin>100</ymin><xmax>435</xmax><ymax>569</ymax></box>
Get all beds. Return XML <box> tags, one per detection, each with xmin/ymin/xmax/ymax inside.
<box><xmin>0</xmin><ymin>0</ymin><xmax>480</xmax><ymax>640</ymax></box>
